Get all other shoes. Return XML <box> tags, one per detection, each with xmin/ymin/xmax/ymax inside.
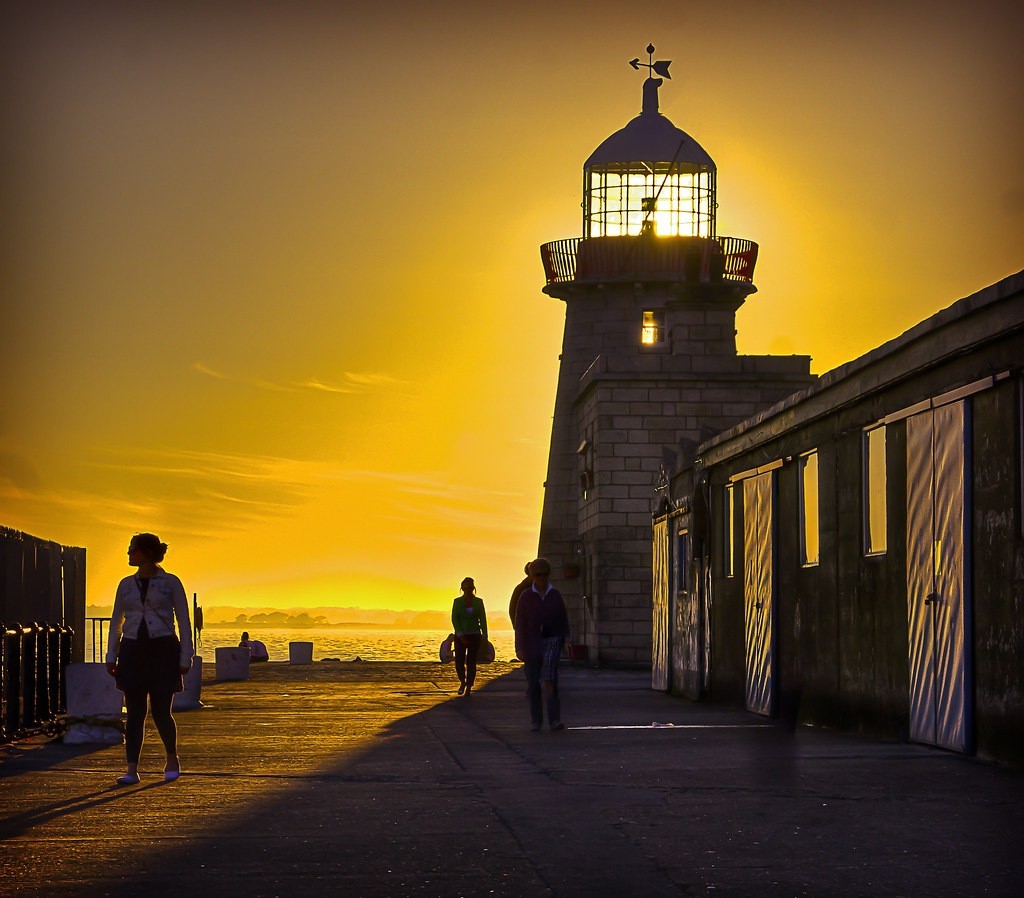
<box><xmin>524</xmin><ymin>689</ymin><xmax>530</xmax><ymax>698</ymax></box>
<box><xmin>529</xmin><ymin>723</ymin><xmax>541</xmax><ymax>731</ymax></box>
<box><xmin>550</xmin><ymin>723</ymin><xmax>564</xmax><ymax>732</ymax></box>
<box><xmin>116</xmin><ymin>772</ymin><xmax>141</xmax><ymax>785</ymax></box>
<box><xmin>458</xmin><ymin>684</ymin><xmax>465</xmax><ymax>694</ymax></box>
<box><xmin>164</xmin><ymin>757</ymin><xmax>179</xmax><ymax>780</ymax></box>
<box><xmin>465</xmin><ymin>688</ymin><xmax>470</xmax><ymax>697</ymax></box>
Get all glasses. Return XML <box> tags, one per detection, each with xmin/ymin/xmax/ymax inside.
<box><xmin>533</xmin><ymin>572</ymin><xmax>549</xmax><ymax>577</ymax></box>
<box><xmin>129</xmin><ymin>546</ymin><xmax>138</xmax><ymax>554</ymax></box>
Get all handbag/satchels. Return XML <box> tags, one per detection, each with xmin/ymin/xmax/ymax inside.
<box><xmin>174</xmin><ymin>636</ymin><xmax>181</xmax><ymax>662</ymax></box>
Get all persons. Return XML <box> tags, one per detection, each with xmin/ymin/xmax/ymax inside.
<box><xmin>238</xmin><ymin>631</ymin><xmax>249</xmax><ymax>647</ymax></box>
<box><xmin>514</xmin><ymin>558</ymin><xmax>571</xmax><ymax>733</ymax></box>
<box><xmin>451</xmin><ymin>577</ymin><xmax>487</xmax><ymax>698</ymax></box>
<box><xmin>439</xmin><ymin>633</ymin><xmax>455</xmax><ymax>664</ymax></box>
<box><xmin>475</xmin><ymin>634</ymin><xmax>495</xmax><ymax>662</ymax></box>
<box><xmin>104</xmin><ymin>532</ymin><xmax>193</xmax><ymax>786</ymax></box>
<box><xmin>509</xmin><ymin>561</ymin><xmax>532</xmax><ymax>629</ymax></box>
